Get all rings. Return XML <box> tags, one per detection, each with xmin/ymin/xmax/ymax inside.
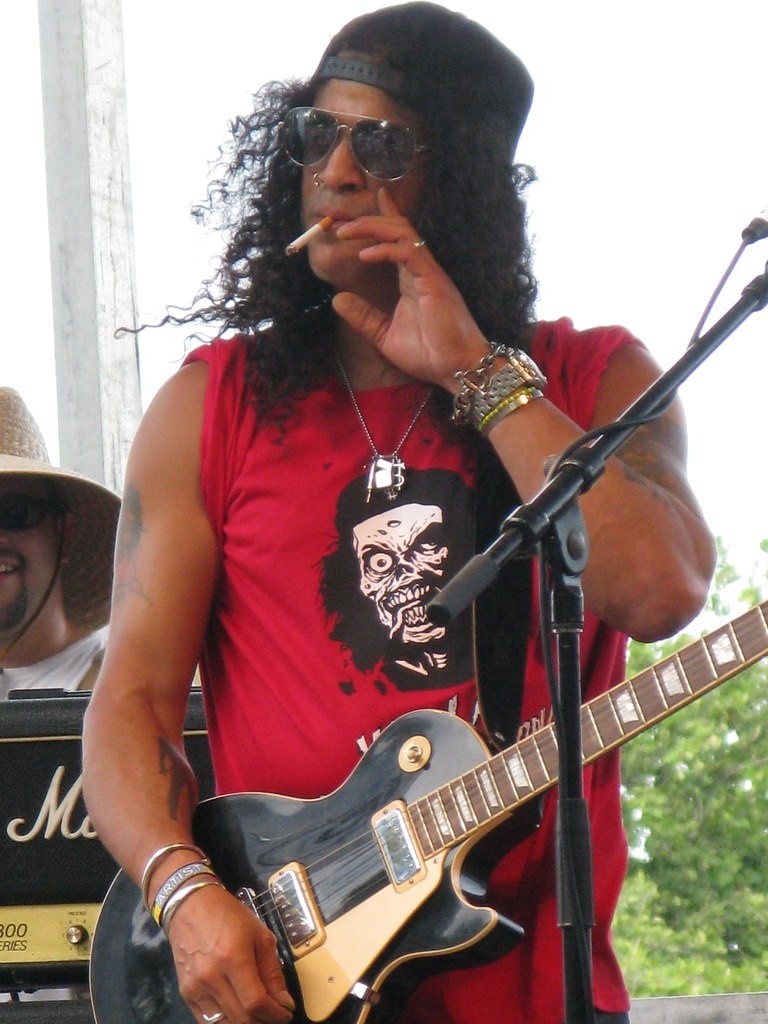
<box><xmin>201</xmin><ymin>1012</ymin><xmax>226</xmax><ymax>1024</ymax></box>
<box><xmin>413</xmin><ymin>240</ymin><xmax>425</xmax><ymax>248</ymax></box>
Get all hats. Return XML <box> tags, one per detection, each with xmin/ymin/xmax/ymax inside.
<box><xmin>0</xmin><ymin>387</ymin><xmax>124</xmax><ymax>634</ymax></box>
<box><xmin>309</xmin><ymin>0</ymin><xmax>535</xmax><ymax>167</ymax></box>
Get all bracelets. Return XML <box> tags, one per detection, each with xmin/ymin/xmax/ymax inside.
<box><xmin>478</xmin><ymin>386</ymin><xmax>545</xmax><ymax>437</ymax></box>
<box><xmin>434</xmin><ymin>342</ymin><xmax>516</xmax><ymax>428</ymax></box>
<box><xmin>162</xmin><ymin>877</ymin><xmax>226</xmax><ymax>939</ymax></box>
<box><xmin>141</xmin><ymin>842</ymin><xmax>211</xmax><ymax>915</ymax></box>
<box><xmin>151</xmin><ymin>861</ymin><xmax>219</xmax><ymax>928</ymax></box>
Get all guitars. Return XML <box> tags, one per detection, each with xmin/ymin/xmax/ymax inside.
<box><xmin>83</xmin><ymin>598</ymin><xmax>768</xmax><ymax>1024</ymax></box>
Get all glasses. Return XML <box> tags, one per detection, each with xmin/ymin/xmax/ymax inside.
<box><xmin>0</xmin><ymin>490</ymin><xmax>58</xmax><ymax>532</ymax></box>
<box><xmin>279</xmin><ymin>107</ymin><xmax>431</xmax><ymax>181</ymax></box>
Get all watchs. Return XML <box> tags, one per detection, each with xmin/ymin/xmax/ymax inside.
<box><xmin>470</xmin><ymin>348</ymin><xmax>548</xmax><ymax>432</ymax></box>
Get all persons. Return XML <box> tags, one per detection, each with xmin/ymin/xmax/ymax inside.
<box><xmin>81</xmin><ymin>1</ymin><xmax>715</xmax><ymax>1024</ymax></box>
<box><xmin>0</xmin><ymin>387</ymin><xmax>123</xmax><ymax>1003</ymax></box>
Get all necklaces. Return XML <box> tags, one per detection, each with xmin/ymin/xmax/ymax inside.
<box><xmin>331</xmin><ymin>342</ymin><xmax>436</xmax><ymax>503</ymax></box>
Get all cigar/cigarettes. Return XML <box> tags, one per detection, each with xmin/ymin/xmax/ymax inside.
<box><xmin>285</xmin><ymin>216</ymin><xmax>335</xmax><ymax>256</ymax></box>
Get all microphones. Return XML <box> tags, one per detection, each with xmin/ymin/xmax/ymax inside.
<box><xmin>742</xmin><ymin>208</ymin><xmax>768</xmax><ymax>245</ymax></box>
